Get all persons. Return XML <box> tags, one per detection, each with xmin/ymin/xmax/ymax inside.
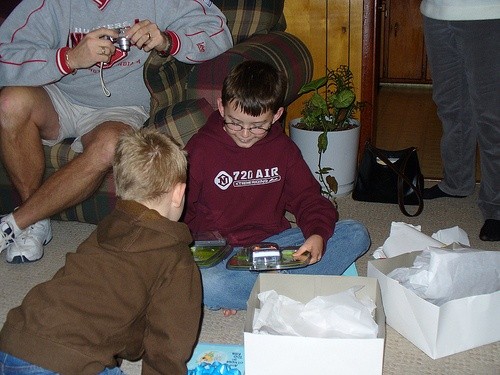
<box><xmin>0</xmin><ymin>0</ymin><xmax>234</xmax><ymax>264</ymax></box>
<box><xmin>420</xmin><ymin>0</ymin><xmax>500</xmax><ymax>241</ymax></box>
<box><xmin>0</xmin><ymin>126</ymin><xmax>203</xmax><ymax>375</ymax></box>
<box><xmin>178</xmin><ymin>60</ymin><xmax>372</xmax><ymax>318</ymax></box>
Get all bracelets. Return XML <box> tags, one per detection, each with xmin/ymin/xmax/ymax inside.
<box><xmin>66</xmin><ymin>47</ymin><xmax>77</xmax><ymax>75</ymax></box>
<box><xmin>155</xmin><ymin>31</ymin><xmax>172</xmax><ymax>57</ymax></box>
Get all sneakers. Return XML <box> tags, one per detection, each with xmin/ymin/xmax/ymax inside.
<box><xmin>0</xmin><ymin>206</ymin><xmax>20</xmax><ymax>253</ymax></box>
<box><xmin>5</xmin><ymin>218</ymin><xmax>53</xmax><ymax>264</ymax></box>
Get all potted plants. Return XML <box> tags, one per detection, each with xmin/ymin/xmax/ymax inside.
<box><xmin>287</xmin><ymin>65</ymin><xmax>372</xmax><ymax>206</ymax></box>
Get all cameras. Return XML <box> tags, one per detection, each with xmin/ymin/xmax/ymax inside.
<box><xmin>108</xmin><ymin>28</ymin><xmax>133</xmax><ymax>52</ymax></box>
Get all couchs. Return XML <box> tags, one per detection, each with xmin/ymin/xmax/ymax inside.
<box><xmin>0</xmin><ymin>0</ymin><xmax>314</xmax><ymax>226</ymax></box>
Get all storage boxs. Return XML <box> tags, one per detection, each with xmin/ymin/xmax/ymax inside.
<box><xmin>243</xmin><ymin>246</ymin><xmax>500</xmax><ymax>375</ymax></box>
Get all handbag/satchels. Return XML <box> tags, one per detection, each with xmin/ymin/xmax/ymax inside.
<box><xmin>352</xmin><ymin>139</ymin><xmax>426</xmax><ymax>217</ymax></box>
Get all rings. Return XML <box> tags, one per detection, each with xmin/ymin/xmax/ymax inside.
<box><xmin>99</xmin><ymin>46</ymin><xmax>105</xmax><ymax>55</ymax></box>
<box><xmin>145</xmin><ymin>32</ymin><xmax>150</xmax><ymax>39</ymax></box>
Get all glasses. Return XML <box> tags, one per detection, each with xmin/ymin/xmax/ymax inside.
<box><xmin>222</xmin><ymin>114</ymin><xmax>274</xmax><ymax>135</ymax></box>
